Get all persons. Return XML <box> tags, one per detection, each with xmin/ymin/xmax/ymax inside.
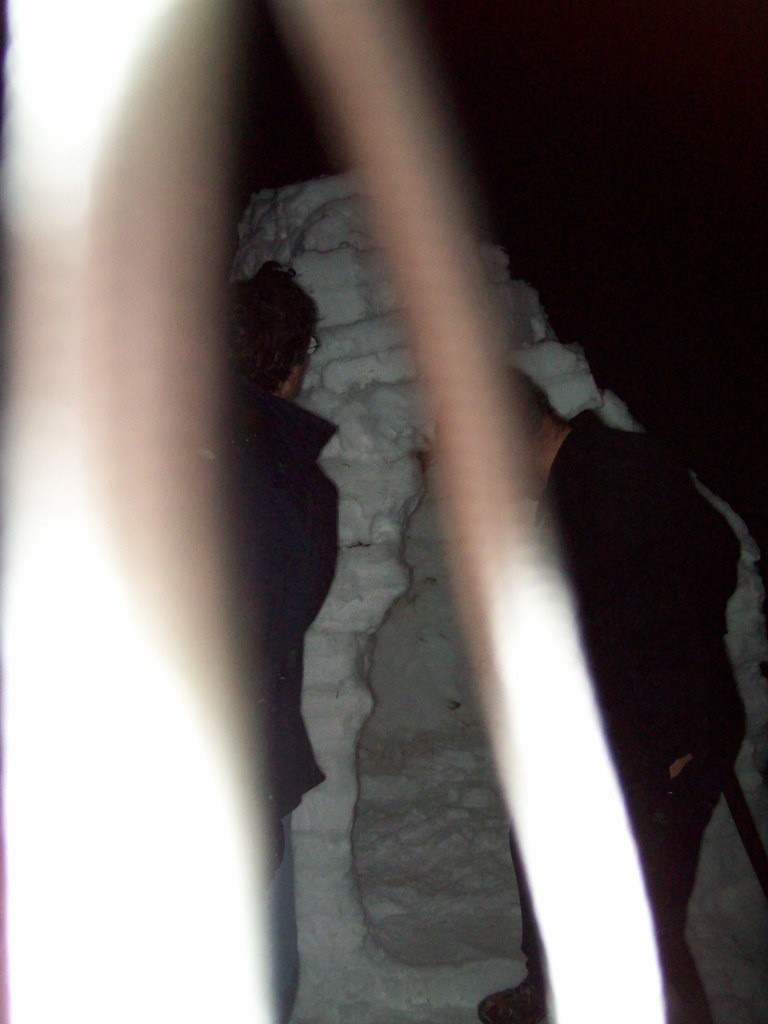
<box><xmin>210</xmin><ymin>254</ymin><xmax>343</xmax><ymax>867</ymax></box>
<box><xmin>476</xmin><ymin>363</ymin><xmax>748</xmax><ymax>1024</ymax></box>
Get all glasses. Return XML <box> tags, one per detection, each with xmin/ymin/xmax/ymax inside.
<box><xmin>301</xmin><ymin>335</ymin><xmax>320</xmax><ymax>356</ymax></box>
